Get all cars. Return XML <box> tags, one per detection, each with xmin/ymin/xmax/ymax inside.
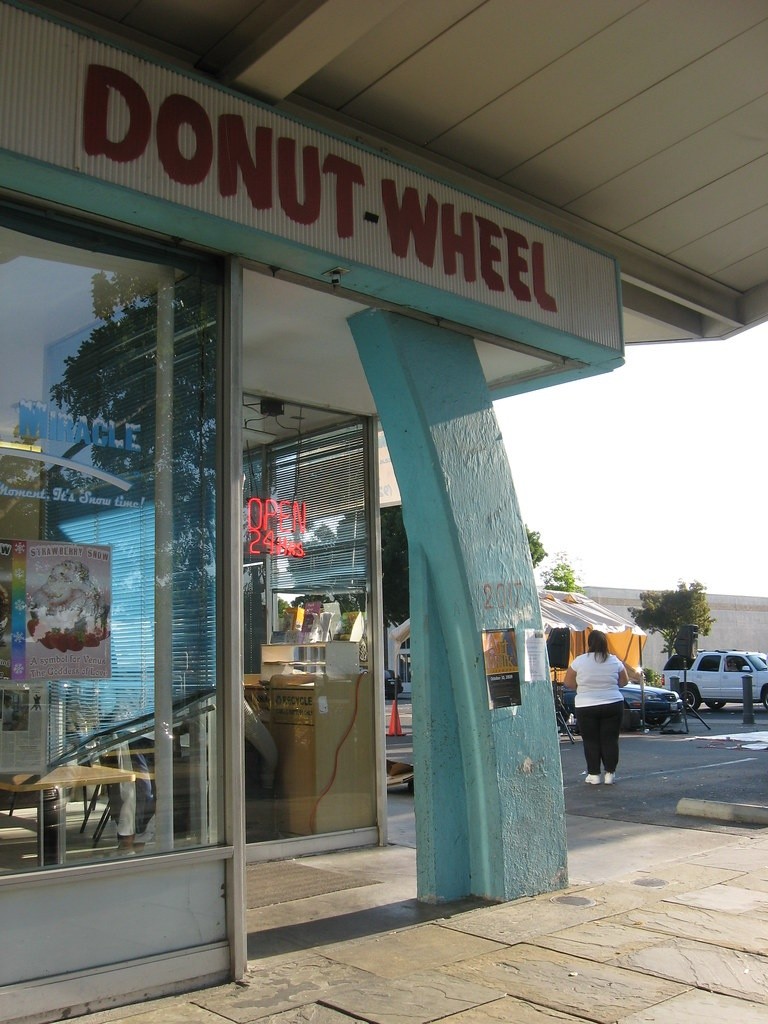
<box><xmin>559</xmin><ymin>680</ymin><xmax>684</xmax><ymax>725</ymax></box>
<box><xmin>383</xmin><ymin>669</ymin><xmax>404</xmax><ymax>698</ymax></box>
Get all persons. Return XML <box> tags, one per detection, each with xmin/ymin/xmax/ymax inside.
<box><xmin>727</xmin><ymin>658</ymin><xmax>739</xmax><ymax>671</ymax></box>
<box><xmin>3</xmin><ymin>694</ymin><xmax>18</xmax><ymax>730</ymax></box>
<box><xmin>564</xmin><ymin>630</ymin><xmax>629</xmax><ymax>785</ymax></box>
<box><xmin>98</xmin><ymin>703</ymin><xmax>156</xmax><ymax>854</ymax></box>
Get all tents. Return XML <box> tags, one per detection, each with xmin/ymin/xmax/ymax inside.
<box><xmin>537</xmin><ymin>589</ymin><xmax>652</xmax><ymax>684</ymax></box>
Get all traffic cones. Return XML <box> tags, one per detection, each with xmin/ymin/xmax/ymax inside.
<box><xmin>385</xmin><ymin>700</ymin><xmax>407</xmax><ymax>736</ymax></box>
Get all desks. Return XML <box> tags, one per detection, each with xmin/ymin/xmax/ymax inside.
<box><xmin>0</xmin><ymin>764</ymin><xmax>135</xmax><ymax>866</ymax></box>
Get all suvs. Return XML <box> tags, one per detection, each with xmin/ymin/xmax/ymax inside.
<box><xmin>660</xmin><ymin>649</ymin><xmax>768</xmax><ymax>709</ymax></box>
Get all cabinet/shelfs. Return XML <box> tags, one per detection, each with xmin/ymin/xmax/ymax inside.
<box><xmin>243</xmin><ymin>640</ymin><xmax>376</xmax><ymax>836</ymax></box>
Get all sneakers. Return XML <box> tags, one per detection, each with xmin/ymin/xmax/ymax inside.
<box><xmin>585</xmin><ymin>774</ymin><xmax>601</xmax><ymax>785</ymax></box>
<box><xmin>604</xmin><ymin>772</ymin><xmax>615</xmax><ymax>785</ymax></box>
